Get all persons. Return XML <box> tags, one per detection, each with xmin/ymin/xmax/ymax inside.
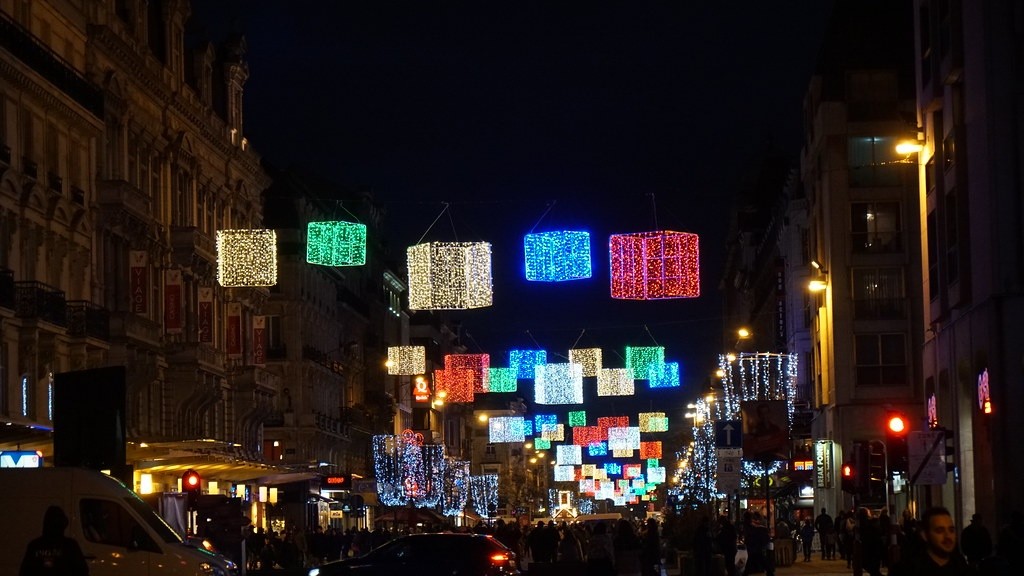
<box><xmin>18</xmin><ymin>505</ymin><xmax>90</xmax><ymax>576</ymax></box>
<box><xmin>245</xmin><ymin>518</ymin><xmax>676</xmax><ymax>576</ymax></box>
<box><xmin>692</xmin><ymin>506</ymin><xmax>1024</xmax><ymax>576</ymax></box>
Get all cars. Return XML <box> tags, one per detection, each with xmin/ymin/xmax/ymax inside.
<box><xmin>575</xmin><ymin>512</ymin><xmax>623</xmax><ymax>542</ymax></box>
<box><xmin>316</xmin><ymin>532</ymin><xmax>521</xmax><ymax>576</ymax></box>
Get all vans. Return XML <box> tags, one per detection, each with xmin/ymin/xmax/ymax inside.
<box><xmin>0</xmin><ymin>465</ymin><xmax>238</xmax><ymax>576</ymax></box>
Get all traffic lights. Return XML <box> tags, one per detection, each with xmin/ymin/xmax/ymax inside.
<box><xmin>840</xmin><ymin>462</ymin><xmax>855</xmax><ymax>492</ymax></box>
<box><xmin>181</xmin><ymin>470</ymin><xmax>201</xmax><ymax>491</ymax></box>
<box><xmin>887</xmin><ymin>412</ymin><xmax>908</xmax><ymax>439</ymax></box>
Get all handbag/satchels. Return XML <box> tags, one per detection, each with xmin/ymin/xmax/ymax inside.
<box><xmin>736</xmin><ymin>523</ymin><xmax>745</xmax><ymax>545</ymax></box>
<box><xmin>846</xmin><ymin>518</ymin><xmax>856</xmax><ymax>530</ymax></box>
<box><xmin>734</xmin><ymin>545</ymin><xmax>748</xmax><ymax>573</ymax></box>
<box><xmin>826</xmin><ymin>534</ymin><xmax>834</xmax><ymax>543</ymax></box>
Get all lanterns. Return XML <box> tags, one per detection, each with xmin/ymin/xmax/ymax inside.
<box><xmin>183</xmin><ymin>468</ymin><xmax>201</xmax><ymax>490</ymax></box>
